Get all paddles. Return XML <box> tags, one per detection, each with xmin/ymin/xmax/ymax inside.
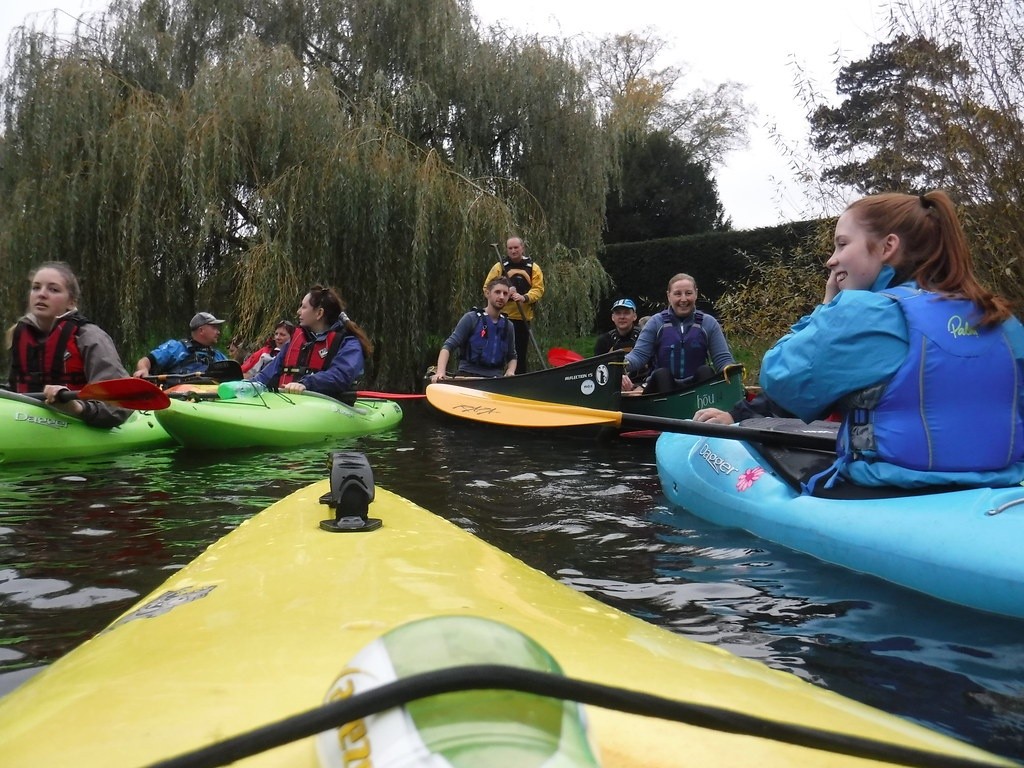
<box><xmin>128</xmin><ymin>360</ymin><xmax>244</xmax><ymax>384</ymax></box>
<box><xmin>490</xmin><ymin>242</ymin><xmax>548</xmax><ymax>371</ymax></box>
<box><xmin>19</xmin><ymin>378</ymin><xmax>172</xmax><ymax>412</ymax></box>
<box><xmin>296</xmin><ymin>390</ymin><xmax>427</xmax><ymax>398</ymax></box>
<box><xmin>546</xmin><ymin>347</ymin><xmax>586</xmax><ymax>369</ymax></box>
<box><xmin>422</xmin><ymin>381</ymin><xmax>836</xmax><ymax>453</ymax></box>
<box><xmin>697</xmin><ymin>415</ymin><xmax>841</xmax><ymax>439</ymax></box>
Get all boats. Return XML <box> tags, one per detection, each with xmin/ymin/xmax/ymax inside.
<box><xmin>623</xmin><ymin>363</ymin><xmax>745</xmax><ymax>436</ymax></box>
<box><xmin>155</xmin><ymin>391</ymin><xmax>403</xmax><ymax>449</ymax></box>
<box><xmin>654</xmin><ymin>421</ymin><xmax>1024</xmax><ymax>621</ymax></box>
<box><xmin>423</xmin><ymin>347</ymin><xmax>624</xmax><ymax>440</ymax></box>
<box><xmin>0</xmin><ymin>396</ymin><xmax>175</xmax><ymax>463</ymax></box>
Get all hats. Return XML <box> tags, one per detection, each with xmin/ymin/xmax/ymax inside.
<box><xmin>189</xmin><ymin>312</ymin><xmax>226</xmax><ymax>329</ymax></box>
<box><xmin>611</xmin><ymin>299</ymin><xmax>636</xmax><ymax>312</ymax></box>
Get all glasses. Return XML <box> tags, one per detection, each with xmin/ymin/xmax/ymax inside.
<box><xmin>279</xmin><ymin>320</ymin><xmax>293</xmax><ymax>328</ymax></box>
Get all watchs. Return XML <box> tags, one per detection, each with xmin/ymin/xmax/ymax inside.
<box><xmin>640</xmin><ymin>383</ymin><xmax>646</xmax><ymax>389</ymax></box>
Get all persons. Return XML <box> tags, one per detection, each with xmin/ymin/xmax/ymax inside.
<box><xmin>692</xmin><ymin>394</ymin><xmax>843</xmax><ymax>482</ymax></box>
<box><xmin>483</xmin><ymin>236</ymin><xmax>544</xmax><ymax>374</ymax></box>
<box><xmin>0</xmin><ymin>261</ymin><xmax>136</xmax><ymax>427</ymax></box>
<box><xmin>133</xmin><ymin>312</ymin><xmax>229</xmax><ymax>389</ymax></box>
<box><xmin>759</xmin><ymin>189</ymin><xmax>1024</xmax><ymax>500</ymax></box>
<box><xmin>249</xmin><ymin>284</ymin><xmax>372</xmax><ymax>406</ymax></box>
<box><xmin>432</xmin><ymin>276</ymin><xmax>517</xmax><ymax>383</ymax></box>
<box><xmin>595</xmin><ymin>299</ymin><xmax>657</xmax><ymax>393</ymax></box>
<box><xmin>620</xmin><ymin>273</ymin><xmax>736</xmax><ymax>395</ymax></box>
<box><xmin>241</xmin><ymin>320</ymin><xmax>295</xmax><ymax>380</ymax></box>
